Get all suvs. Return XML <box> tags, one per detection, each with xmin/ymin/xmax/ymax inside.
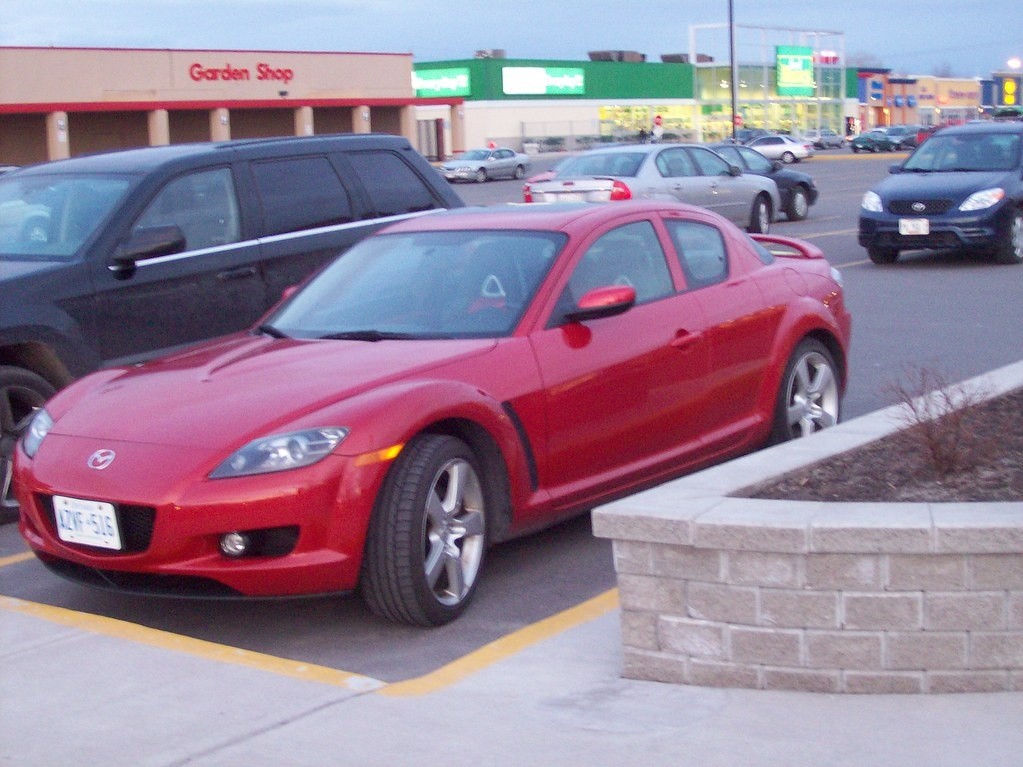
<box><xmin>0</xmin><ymin>131</ymin><xmax>483</xmax><ymax>523</ymax></box>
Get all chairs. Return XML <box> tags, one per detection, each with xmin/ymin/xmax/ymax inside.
<box><xmin>409</xmin><ymin>245</ymin><xmax>480</xmax><ymax>327</ymax></box>
<box><xmin>956</xmin><ymin>142</ymin><xmax>977</xmax><ymax>169</ymax></box>
<box><xmin>618</xmin><ymin>161</ymin><xmax>639</xmax><ymax>176</ymax></box>
<box><xmin>667</xmin><ymin>158</ymin><xmax>687</xmax><ymax>177</ymax></box>
<box><xmin>980</xmin><ymin>143</ymin><xmax>1004</xmax><ymax>168</ymax></box>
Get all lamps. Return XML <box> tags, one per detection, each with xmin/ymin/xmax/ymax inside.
<box><xmin>278</xmin><ymin>89</ymin><xmax>289</xmax><ymax>99</ymax></box>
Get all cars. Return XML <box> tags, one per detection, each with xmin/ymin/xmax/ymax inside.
<box><xmin>856</xmin><ymin>118</ymin><xmax>1023</xmax><ymax>264</ymax></box>
<box><xmin>722</xmin><ymin>123</ymin><xmax>940</xmax><ymax>165</ymax></box>
<box><xmin>524</xmin><ymin>142</ymin><xmax>783</xmax><ymax>236</ymax></box>
<box><xmin>15</xmin><ymin>198</ymin><xmax>852</xmax><ymax>630</ymax></box>
<box><xmin>440</xmin><ymin>147</ymin><xmax>530</xmax><ymax>183</ymax></box>
<box><xmin>693</xmin><ymin>142</ymin><xmax>822</xmax><ymax>221</ymax></box>
